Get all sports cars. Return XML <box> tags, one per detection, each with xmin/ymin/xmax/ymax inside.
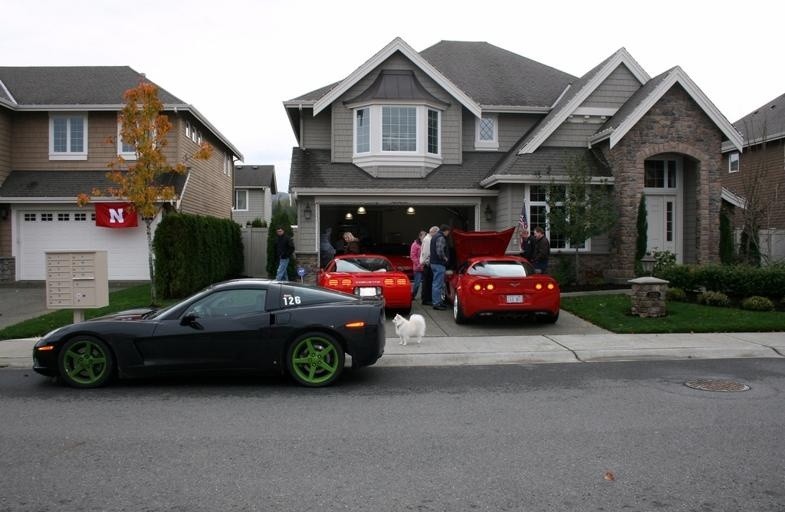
<box><xmin>444</xmin><ymin>226</ymin><xmax>560</xmax><ymax>324</ymax></box>
<box><xmin>315</xmin><ymin>242</ymin><xmax>415</xmax><ymax>316</ymax></box>
<box><xmin>33</xmin><ymin>278</ymin><xmax>387</xmax><ymax>388</ymax></box>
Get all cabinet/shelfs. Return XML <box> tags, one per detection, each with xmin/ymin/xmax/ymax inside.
<box><xmin>44</xmin><ymin>249</ymin><xmax>110</xmax><ymax>310</ymax></box>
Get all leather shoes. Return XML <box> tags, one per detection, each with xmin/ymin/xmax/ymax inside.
<box><xmin>422</xmin><ymin>300</ymin><xmax>447</xmax><ymax>310</ymax></box>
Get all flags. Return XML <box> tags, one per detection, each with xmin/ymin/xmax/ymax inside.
<box><xmin>93</xmin><ymin>200</ymin><xmax>139</xmax><ymax>228</ymax></box>
<box><xmin>514</xmin><ymin>198</ymin><xmax>529</xmax><ymax>254</ymax></box>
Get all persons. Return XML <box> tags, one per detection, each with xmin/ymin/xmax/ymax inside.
<box><xmin>430</xmin><ymin>224</ymin><xmax>452</xmax><ymax>310</ymax></box>
<box><xmin>419</xmin><ymin>225</ymin><xmax>440</xmax><ymax>306</ymax></box>
<box><xmin>342</xmin><ymin>231</ymin><xmax>361</xmax><ymax>254</ymax></box>
<box><xmin>529</xmin><ymin>226</ymin><xmax>551</xmax><ymax>275</ymax></box>
<box><xmin>514</xmin><ymin>228</ymin><xmax>537</xmax><ymax>262</ymax></box>
<box><xmin>410</xmin><ymin>229</ymin><xmax>427</xmax><ymax>300</ymax></box>
<box><xmin>320</xmin><ymin>226</ymin><xmax>337</xmax><ymax>269</ymax></box>
<box><xmin>273</xmin><ymin>226</ymin><xmax>296</xmax><ymax>282</ymax></box>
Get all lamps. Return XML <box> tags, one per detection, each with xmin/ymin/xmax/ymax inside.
<box><xmin>344</xmin><ymin>207</ymin><xmax>354</xmax><ymax>220</ymax></box>
<box><xmin>485</xmin><ymin>202</ymin><xmax>493</xmax><ymax>221</ymax></box>
<box><xmin>406</xmin><ymin>206</ymin><xmax>416</xmax><ymax>216</ymax></box>
<box><xmin>303</xmin><ymin>202</ymin><xmax>312</xmax><ymax>219</ymax></box>
<box><xmin>356</xmin><ymin>203</ymin><xmax>367</xmax><ymax>215</ymax></box>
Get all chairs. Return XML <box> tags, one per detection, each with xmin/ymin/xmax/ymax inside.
<box><xmin>255</xmin><ymin>294</ymin><xmax>265</xmax><ymax>311</ymax></box>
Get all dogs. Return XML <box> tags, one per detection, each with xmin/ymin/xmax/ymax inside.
<box><xmin>391</xmin><ymin>313</ymin><xmax>425</xmax><ymax>346</ymax></box>
